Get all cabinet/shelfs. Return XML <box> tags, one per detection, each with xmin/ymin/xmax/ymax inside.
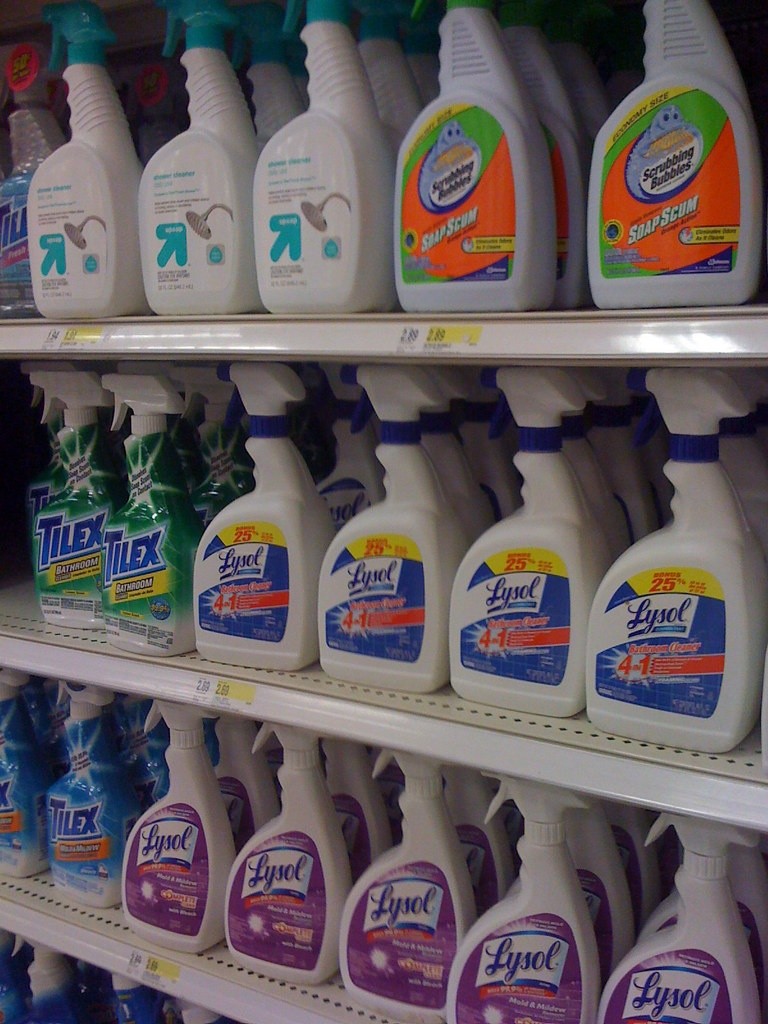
<box><xmin>0</xmin><ymin>0</ymin><xmax>768</xmax><ymax>1024</ymax></box>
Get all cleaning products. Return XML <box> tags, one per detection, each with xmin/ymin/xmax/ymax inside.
<box><xmin>18</xmin><ymin>358</ymin><xmax>767</xmax><ymax>757</ymax></box>
<box><xmin>0</xmin><ymin>0</ymin><xmax>766</xmax><ymax>321</ymax></box>
<box><xmin>0</xmin><ymin>665</ymin><xmax>768</xmax><ymax>1024</ymax></box>
